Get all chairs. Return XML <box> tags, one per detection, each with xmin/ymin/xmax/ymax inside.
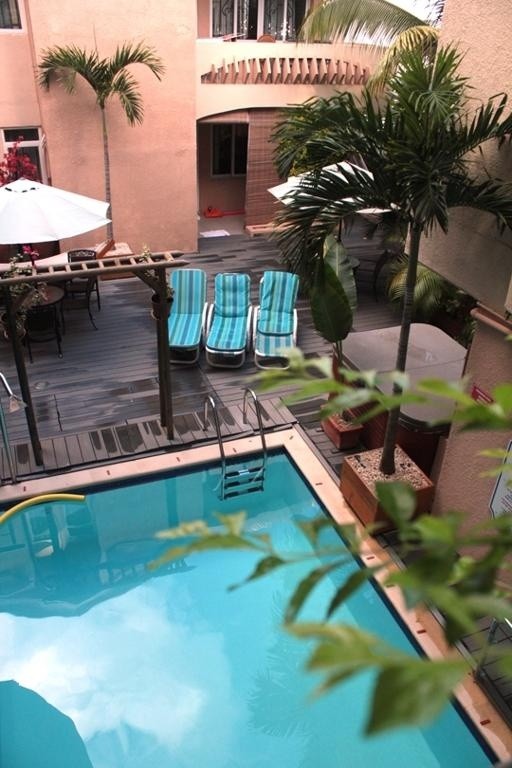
<box><xmin>252</xmin><ymin>271</ymin><xmax>300</xmax><ymax>371</ymax></box>
<box><xmin>166</xmin><ymin>269</ymin><xmax>209</xmax><ymax>365</ymax></box>
<box><xmin>204</xmin><ymin>272</ymin><xmax>251</xmax><ymax>368</ymax></box>
<box><xmin>67</xmin><ymin>249</ymin><xmax>101</xmax><ymax>312</ymax></box>
<box><xmin>59</xmin><ymin>276</ymin><xmax>98</xmax><ymax>336</ymax></box>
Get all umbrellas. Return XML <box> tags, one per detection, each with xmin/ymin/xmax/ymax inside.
<box><xmin>266</xmin><ymin>159</ymin><xmax>403</xmax><ymax>242</ymax></box>
<box><xmin>0</xmin><ymin>176</ymin><xmax>113</xmax><ymax>266</ymax></box>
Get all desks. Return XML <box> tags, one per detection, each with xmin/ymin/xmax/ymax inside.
<box><xmin>21</xmin><ymin>285</ymin><xmax>65</xmax><ymax>364</ymax></box>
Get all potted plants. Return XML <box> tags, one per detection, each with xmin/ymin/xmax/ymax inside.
<box><xmin>309</xmin><ymin>233</ymin><xmax>365</xmax><ymax>450</ymax></box>
<box><xmin>266</xmin><ymin>26</ymin><xmax>511</xmax><ymax>538</ymax></box>
<box><xmin>35</xmin><ymin>23</ymin><xmax>166</xmax><ymax>280</ymax></box>
